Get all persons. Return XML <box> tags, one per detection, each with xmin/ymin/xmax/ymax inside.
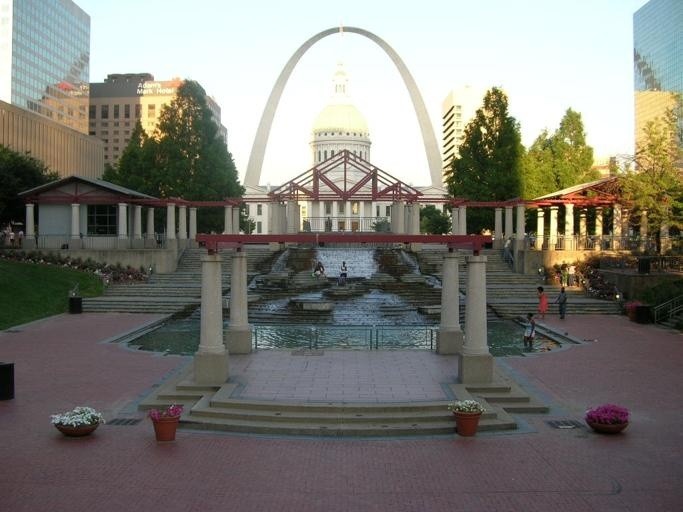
<box><xmin>553</xmin><ymin>287</ymin><xmax>567</xmax><ymax>319</ymax></box>
<box><xmin>18</xmin><ymin>229</ymin><xmax>24</xmax><ymax>248</ymax></box>
<box><xmin>9</xmin><ymin>230</ymin><xmax>15</xmax><ymax>248</ymax></box>
<box><xmin>536</xmin><ymin>287</ymin><xmax>548</xmax><ymax>319</ymax></box>
<box><xmin>503</xmin><ymin>236</ymin><xmax>513</xmax><ymax>264</ymax></box>
<box><xmin>340</xmin><ymin>261</ymin><xmax>348</xmax><ymax>277</ymax></box>
<box><xmin>524</xmin><ymin>313</ymin><xmax>536</xmax><ymax>347</ymax></box>
<box><xmin>314</xmin><ymin>262</ymin><xmax>324</xmax><ymax>273</ymax></box>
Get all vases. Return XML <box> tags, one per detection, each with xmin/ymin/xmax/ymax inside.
<box><xmin>151</xmin><ymin>418</ymin><xmax>177</xmax><ymax>443</ymax></box>
<box><xmin>0</xmin><ymin>360</ymin><xmax>17</xmax><ymax>400</ymax></box>
<box><xmin>634</xmin><ymin>305</ymin><xmax>652</xmax><ymax>325</ymax></box>
<box><xmin>591</xmin><ymin>421</ymin><xmax>626</xmax><ymax>435</ymax></box>
<box><xmin>453</xmin><ymin>411</ymin><xmax>483</xmax><ymax>436</ymax></box>
<box><xmin>67</xmin><ymin>295</ymin><xmax>83</xmax><ymax>312</ymax></box>
<box><xmin>55</xmin><ymin>424</ymin><xmax>100</xmax><ymax>436</ymax></box>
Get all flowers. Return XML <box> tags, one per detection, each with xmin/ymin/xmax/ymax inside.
<box><xmin>585</xmin><ymin>403</ymin><xmax>631</xmax><ymax>426</ymax></box>
<box><xmin>449</xmin><ymin>398</ymin><xmax>488</xmax><ymax>418</ymax></box>
<box><xmin>147</xmin><ymin>404</ymin><xmax>183</xmax><ymax>421</ymax></box>
<box><xmin>48</xmin><ymin>404</ymin><xmax>107</xmax><ymax>430</ymax></box>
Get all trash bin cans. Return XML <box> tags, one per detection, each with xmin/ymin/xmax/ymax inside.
<box><xmin>69</xmin><ymin>296</ymin><xmax>82</xmax><ymax>314</ymax></box>
<box><xmin>0</xmin><ymin>361</ymin><xmax>15</xmax><ymax>400</ymax></box>
<box><xmin>637</xmin><ymin>305</ymin><xmax>653</xmax><ymax>324</ymax></box>
